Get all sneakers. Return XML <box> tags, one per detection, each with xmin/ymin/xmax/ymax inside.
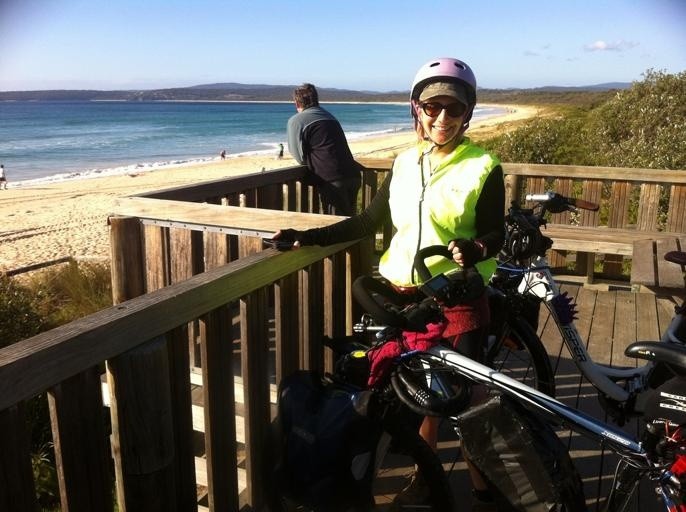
<box><xmin>393</xmin><ymin>471</ymin><xmax>430</xmax><ymax>505</ymax></box>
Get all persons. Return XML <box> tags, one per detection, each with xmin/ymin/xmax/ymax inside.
<box><xmin>285</xmin><ymin>83</ymin><xmax>362</xmax><ymax>284</ymax></box>
<box><xmin>220</xmin><ymin>149</ymin><xmax>225</xmax><ymax>160</ymax></box>
<box><xmin>277</xmin><ymin>143</ymin><xmax>284</xmax><ymax>160</ymax></box>
<box><xmin>0</xmin><ymin>164</ymin><xmax>8</xmax><ymax>190</ymax></box>
<box><xmin>270</xmin><ymin>59</ymin><xmax>504</xmax><ymax>507</ymax></box>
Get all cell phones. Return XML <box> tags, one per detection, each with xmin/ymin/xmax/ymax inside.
<box><xmin>263</xmin><ymin>237</ymin><xmax>296</xmax><ymax>247</ymax></box>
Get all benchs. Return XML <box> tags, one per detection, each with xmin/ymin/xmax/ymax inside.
<box><xmin>630</xmin><ymin>238</ymin><xmax>685</xmax><ymax>288</ymax></box>
<box><xmin>539</xmin><ymin>226</ymin><xmax>679</xmax><ymax>283</ymax></box>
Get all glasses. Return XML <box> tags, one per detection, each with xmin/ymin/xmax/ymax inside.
<box><xmin>416</xmin><ymin>99</ymin><xmax>466</xmax><ymax>117</ymax></box>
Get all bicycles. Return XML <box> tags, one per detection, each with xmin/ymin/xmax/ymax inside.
<box><xmin>473</xmin><ymin>195</ymin><xmax>686</xmax><ymax>428</ymax></box>
<box><xmin>283</xmin><ymin>246</ymin><xmax>686</xmax><ymax>512</ymax></box>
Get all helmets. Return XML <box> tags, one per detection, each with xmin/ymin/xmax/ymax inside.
<box><xmin>409</xmin><ymin>59</ymin><xmax>477</xmax><ymax>101</ymax></box>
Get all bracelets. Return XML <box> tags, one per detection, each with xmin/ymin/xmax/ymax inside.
<box><xmin>474</xmin><ymin>239</ymin><xmax>488</xmax><ymax>261</ymax></box>
